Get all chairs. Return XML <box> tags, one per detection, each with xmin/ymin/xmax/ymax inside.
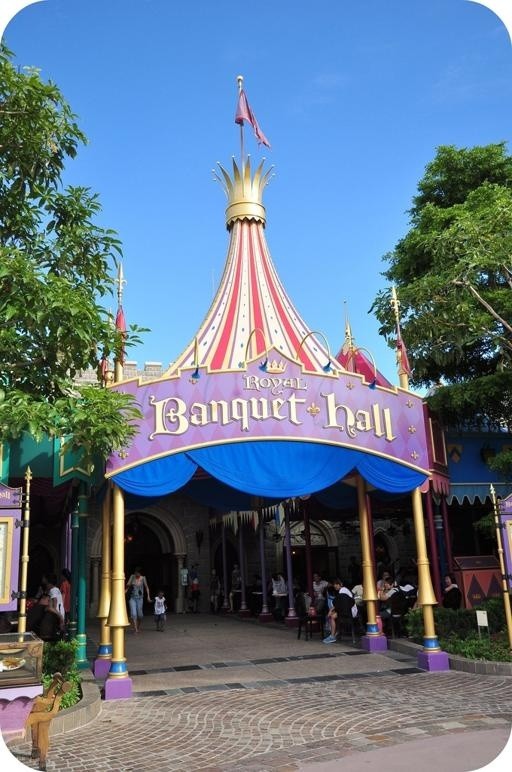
<box><xmin>295</xmin><ymin>589</ymin><xmax>417</xmax><ymax>642</ymax></box>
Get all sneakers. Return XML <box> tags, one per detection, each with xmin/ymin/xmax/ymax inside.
<box><xmin>322</xmin><ymin>634</ymin><xmax>337</xmax><ymax>643</ymax></box>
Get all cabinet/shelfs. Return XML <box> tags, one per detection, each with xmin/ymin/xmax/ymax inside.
<box><xmin>0</xmin><ymin>632</ymin><xmax>45</xmax><ymax>763</ymax></box>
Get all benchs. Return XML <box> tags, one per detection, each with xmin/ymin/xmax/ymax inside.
<box><xmin>0</xmin><ymin>671</ymin><xmax>73</xmax><ymax>771</ymax></box>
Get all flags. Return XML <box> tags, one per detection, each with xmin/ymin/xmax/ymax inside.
<box><xmin>234</xmin><ymin>90</ymin><xmax>268</xmax><ymax>148</ymax></box>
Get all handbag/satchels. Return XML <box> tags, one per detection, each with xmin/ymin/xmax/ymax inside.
<box><xmin>126</xmin><ymin>585</ymin><xmax>133</xmax><ymax>600</ymax></box>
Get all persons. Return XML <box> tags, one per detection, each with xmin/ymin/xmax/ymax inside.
<box><xmin>26</xmin><ymin>565</ymin><xmax>73</xmax><ymax>644</ymax></box>
<box><xmin>125</xmin><ymin>565</ymin><xmax>150</xmax><ymax>636</ymax></box>
<box><xmin>186</xmin><ymin>567</ymin><xmax>464</xmax><ymax>647</ymax></box>
<box><xmin>149</xmin><ymin>592</ymin><xmax>168</xmax><ymax>633</ymax></box>
